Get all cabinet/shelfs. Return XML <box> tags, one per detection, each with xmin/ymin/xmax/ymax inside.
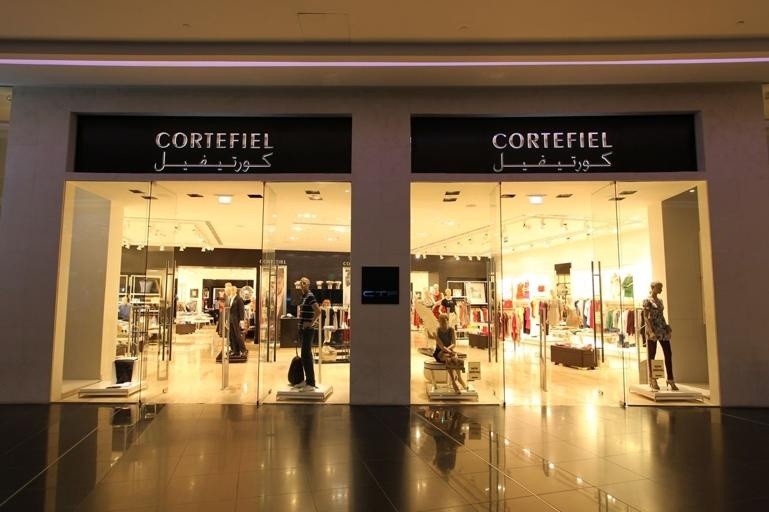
<box><xmin>119</xmin><ymin>275</ymin><xmax>350</xmax><ymax>364</ymax></box>
<box><xmin>412</xmin><ymin>274</ymin><xmax>640</xmax><ymax>365</ymax></box>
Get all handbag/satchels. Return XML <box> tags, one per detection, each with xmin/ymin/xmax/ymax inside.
<box><xmin>288</xmin><ymin>356</ymin><xmax>304</xmax><ymax>384</ymax></box>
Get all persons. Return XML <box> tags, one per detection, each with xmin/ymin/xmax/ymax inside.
<box><xmin>544</xmin><ymin>287</ymin><xmax>562</xmax><ymax>326</ymax></box>
<box><xmin>640</xmin><ymin>280</ymin><xmax>679</xmax><ymax>392</ymax></box>
<box><xmin>223</xmin><ymin>285</ymin><xmax>255</xmax><ymax>360</ymax></box>
<box><xmin>214</xmin><ymin>296</ymin><xmax>224</xmax><ymax>315</ymax></box>
<box><xmin>117</xmin><ymin>296</ymin><xmax>131</xmax><ymax>322</ymax></box>
<box><xmin>564</xmin><ymin>295</ymin><xmax>578</xmax><ymax>326</ymax></box>
<box><xmin>319</xmin><ymin>300</ymin><xmax>336</xmax><ymax>344</ymax></box>
<box><xmin>432</xmin><ymin>313</ymin><xmax>469</xmax><ymax>395</ymax></box>
<box><xmin>431</xmin><ymin>284</ymin><xmax>458</xmax><ymax>319</ymax></box>
<box><xmin>295</xmin><ymin>277</ymin><xmax>323</xmax><ymax>393</ymax></box>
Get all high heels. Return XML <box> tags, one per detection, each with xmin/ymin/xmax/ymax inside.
<box><xmin>666</xmin><ymin>379</ymin><xmax>678</xmax><ymax>390</ymax></box>
<box><xmin>650</xmin><ymin>378</ymin><xmax>660</xmax><ymax>389</ymax></box>
